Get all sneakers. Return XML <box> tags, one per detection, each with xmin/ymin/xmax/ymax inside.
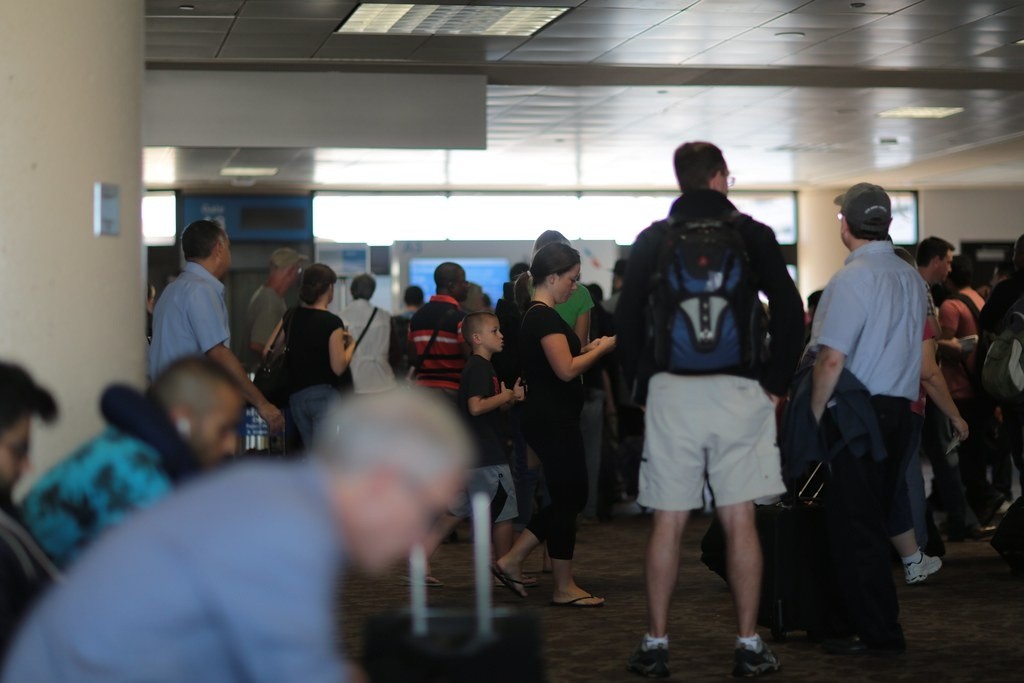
<box><xmin>903</xmin><ymin>552</ymin><xmax>945</xmax><ymax>587</ymax></box>
<box><xmin>624</xmin><ymin>642</ymin><xmax>673</xmax><ymax>679</ymax></box>
<box><xmin>730</xmin><ymin>637</ymin><xmax>783</xmax><ymax>679</ymax></box>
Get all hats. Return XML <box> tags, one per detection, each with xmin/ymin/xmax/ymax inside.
<box><xmin>835</xmin><ymin>182</ymin><xmax>895</xmax><ymax>234</ymax></box>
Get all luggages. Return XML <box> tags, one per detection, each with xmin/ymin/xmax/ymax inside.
<box><xmin>365</xmin><ymin>479</ymin><xmax>546</xmax><ymax>683</ymax></box>
<box><xmin>698</xmin><ymin>458</ymin><xmax>835</xmax><ymax>602</ymax></box>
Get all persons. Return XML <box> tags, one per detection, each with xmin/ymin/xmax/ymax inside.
<box><xmin>17</xmin><ymin>352</ymin><xmax>240</xmax><ymax>573</ymax></box>
<box><xmin>492</xmin><ymin>229</ymin><xmax>606</xmax><ymax>607</ymax></box>
<box><xmin>398</xmin><ymin>286</ymin><xmax>425</xmax><ymax>379</ymax></box>
<box><xmin>406</xmin><ymin>262</ymin><xmax>473</xmax><ymax>543</ymax></box>
<box><xmin>148</xmin><ymin>221</ymin><xmax>285</xmax><ymax>437</ymax></box>
<box><xmin>893</xmin><ymin>234</ymin><xmax>1024</xmax><ymax>584</ymax></box>
<box><xmin>580</xmin><ymin>256</ymin><xmax>649</xmax><ymax>525</ymax></box>
<box><xmin>810</xmin><ymin>183</ymin><xmax>929</xmax><ymax>656</ymax></box>
<box><xmin>424</xmin><ymin>312</ymin><xmax>541</xmax><ymax>587</ymax></box>
<box><xmin>0</xmin><ymin>360</ymin><xmax>71</xmax><ymax>683</ymax></box>
<box><xmin>336</xmin><ymin>273</ymin><xmax>397</xmax><ymax>395</ymax></box>
<box><xmin>0</xmin><ymin>381</ymin><xmax>478</xmax><ymax>683</ymax></box>
<box><xmin>609</xmin><ymin>141</ymin><xmax>805</xmax><ymax>680</ymax></box>
<box><xmin>262</xmin><ymin>264</ymin><xmax>355</xmax><ymax>452</ymax></box>
<box><xmin>247</xmin><ymin>249</ymin><xmax>300</xmax><ymax>354</ymax></box>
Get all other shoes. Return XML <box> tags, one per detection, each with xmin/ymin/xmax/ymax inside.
<box><xmin>949</xmin><ymin>523</ymin><xmax>998</xmax><ymax>542</ymax></box>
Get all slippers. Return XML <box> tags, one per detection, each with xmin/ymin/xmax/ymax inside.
<box><xmin>400</xmin><ymin>574</ymin><xmax>445</xmax><ymax>589</ymax></box>
<box><xmin>491</xmin><ymin>565</ymin><xmax>525</xmax><ymax>597</ymax></box>
<box><xmin>493</xmin><ymin>578</ymin><xmax>538</xmax><ymax>587</ymax></box>
<box><xmin>553</xmin><ymin>591</ymin><xmax>603</xmax><ymax>609</ymax></box>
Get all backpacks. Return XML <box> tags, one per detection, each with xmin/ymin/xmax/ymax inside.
<box><xmin>656</xmin><ymin>208</ymin><xmax>754</xmax><ymax>376</ymax></box>
<box><xmin>977</xmin><ymin>295</ymin><xmax>1023</xmax><ymax>402</ymax></box>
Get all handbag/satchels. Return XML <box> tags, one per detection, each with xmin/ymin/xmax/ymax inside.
<box><xmin>251</xmin><ymin>356</ymin><xmax>289</xmax><ymax>400</ymax></box>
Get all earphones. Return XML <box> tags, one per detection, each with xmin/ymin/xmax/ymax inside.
<box><xmin>178</xmin><ymin>419</ymin><xmax>192</xmax><ymax>438</ymax></box>
